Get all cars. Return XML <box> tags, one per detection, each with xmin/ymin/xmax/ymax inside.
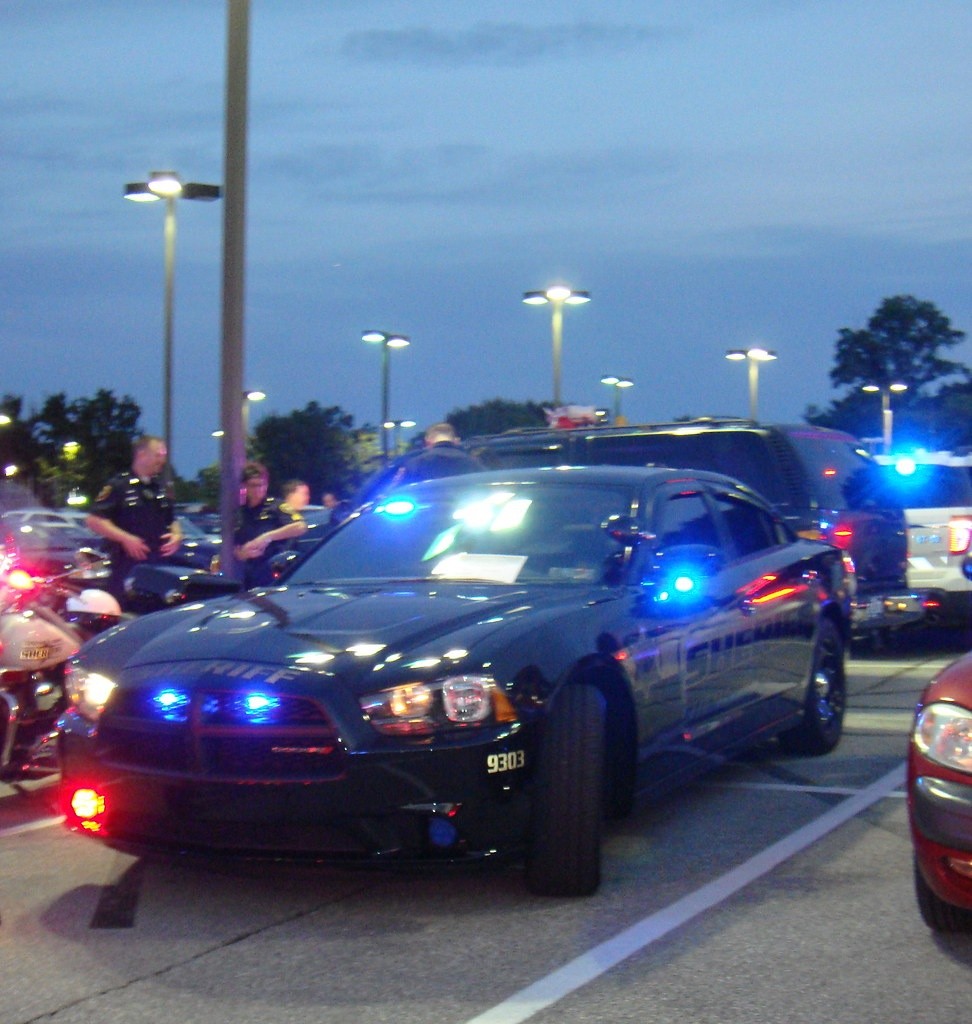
<box><xmin>0</xmin><ymin>503</ymin><xmax>225</xmax><ymax>569</ymax></box>
<box><xmin>51</xmin><ymin>472</ymin><xmax>856</xmax><ymax>906</ymax></box>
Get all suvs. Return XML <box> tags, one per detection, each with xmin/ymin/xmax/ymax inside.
<box><xmin>269</xmin><ymin>416</ymin><xmax>906</xmax><ymax>642</ymax></box>
<box><xmin>856</xmin><ymin>450</ymin><xmax>971</xmax><ymax>648</ymax></box>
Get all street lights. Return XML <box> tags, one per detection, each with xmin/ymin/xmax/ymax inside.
<box><xmin>861</xmin><ymin>381</ymin><xmax>912</xmax><ymax>450</ymax></box>
<box><xmin>382</xmin><ymin>417</ymin><xmax>417</xmax><ymax>456</ymax></box>
<box><xmin>600</xmin><ymin>373</ymin><xmax>634</xmax><ymax>425</ymax></box>
<box><xmin>722</xmin><ymin>345</ymin><xmax>780</xmax><ymax>426</ymax></box>
<box><xmin>241</xmin><ymin>391</ymin><xmax>266</xmax><ymax>440</ymax></box>
<box><xmin>121</xmin><ymin>168</ymin><xmax>227</xmax><ymax>504</ymax></box>
<box><xmin>358</xmin><ymin>330</ymin><xmax>412</xmax><ymax>476</ymax></box>
<box><xmin>524</xmin><ymin>285</ymin><xmax>592</xmax><ymax>417</ymax></box>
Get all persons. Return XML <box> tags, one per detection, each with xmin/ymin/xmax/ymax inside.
<box><xmin>227</xmin><ymin>460</ymin><xmax>308</xmax><ymax>590</ymax></box>
<box><xmin>85</xmin><ymin>439</ymin><xmax>183</xmax><ymax>612</ymax></box>
<box><xmin>274</xmin><ymin>475</ymin><xmax>310</xmax><ymax>514</ymax></box>
<box><xmin>324</xmin><ymin>492</ymin><xmax>341</xmax><ymax>508</ymax></box>
<box><xmin>468</xmin><ymin>444</ymin><xmax>506</xmax><ymax>474</ymax></box>
<box><xmin>390</xmin><ymin>423</ymin><xmax>490</xmax><ymax>486</ymax></box>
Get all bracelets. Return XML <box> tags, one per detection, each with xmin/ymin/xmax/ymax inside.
<box><xmin>263</xmin><ymin>533</ymin><xmax>273</xmax><ymax>543</ymax></box>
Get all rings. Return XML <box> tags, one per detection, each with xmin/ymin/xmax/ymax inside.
<box><xmin>252</xmin><ymin>548</ymin><xmax>258</xmax><ymax>553</ymax></box>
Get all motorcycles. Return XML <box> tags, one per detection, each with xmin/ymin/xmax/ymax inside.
<box><xmin>0</xmin><ymin>544</ymin><xmax>240</xmax><ymax>800</ymax></box>
